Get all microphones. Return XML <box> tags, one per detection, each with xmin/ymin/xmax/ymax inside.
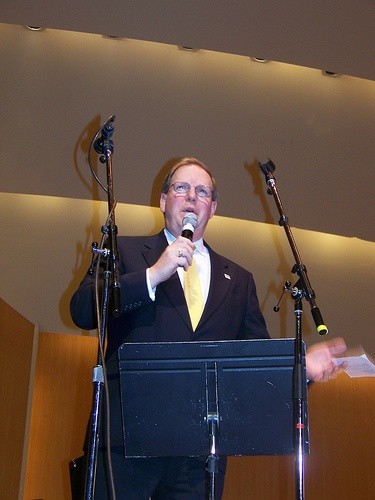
<box><xmin>184</xmin><ymin>212</ymin><xmax>198</xmax><ymax>242</ymax></box>
<box><xmin>95</xmin><ymin>115</ymin><xmax>115</xmax><ymax>154</ymax></box>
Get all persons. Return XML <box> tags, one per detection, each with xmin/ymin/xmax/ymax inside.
<box><xmin>70</xmin><ymin>158</ymin><xmax>349</xmax><ymax>500</ymax></box>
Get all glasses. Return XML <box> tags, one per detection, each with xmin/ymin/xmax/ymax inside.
<box><xmin>165</xmin><ymin>182</ymin><xmax>213</xmax><ymax>197</ymax></box>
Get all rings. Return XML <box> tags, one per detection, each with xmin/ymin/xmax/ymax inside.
<box><xmin>178</xmin><ymin>248</ymin><xmax>183</xmax><ymax>258</ymax></box>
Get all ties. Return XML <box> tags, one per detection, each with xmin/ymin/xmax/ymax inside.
<box><xmin>185</xmin><ymin>246</ymin><xmax>203</xmax><ymax>332</ymax></box>
